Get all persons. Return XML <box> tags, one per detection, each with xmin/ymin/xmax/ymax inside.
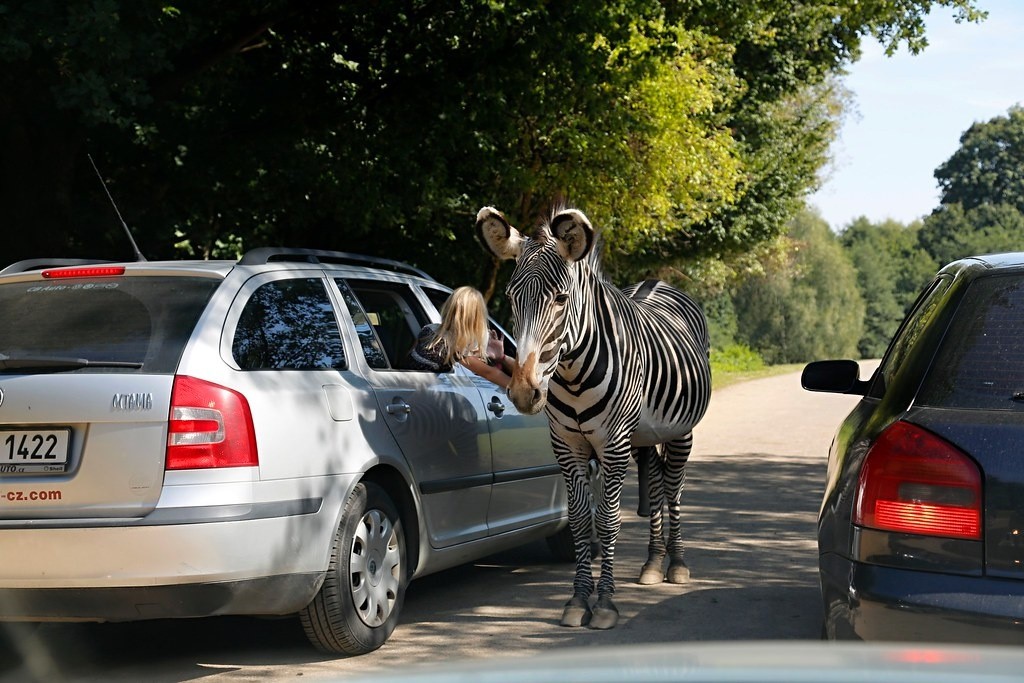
<box><xmin>486</xmin><ymin>329</ymin><xmax>515</xmax><ymax>375</ymax></box>
<box><xmin>401</xmin><ymin>287</ymin><xmax>511</xmax><ymax>387</ymax></box>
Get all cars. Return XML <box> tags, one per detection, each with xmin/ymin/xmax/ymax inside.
<box><xmin>0</xmin><ymin>243</ymin><xmax>602</xmax><ymax>657</ymax></box>
<box><xmin>796</xmin><ymin>251</ymin><xmax>1024</xmax><ymax>648</ymax></box>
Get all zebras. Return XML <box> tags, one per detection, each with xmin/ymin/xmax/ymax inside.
<box><xmin>473</xmin><ymin>205</ymin><xmax>713</xmax><ymax>628</ymax></box>
